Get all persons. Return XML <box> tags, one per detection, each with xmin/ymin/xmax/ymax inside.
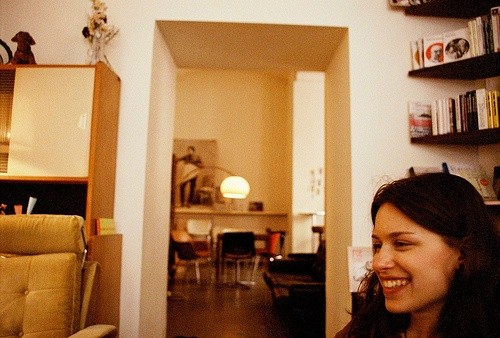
<box><xmin>330</xmin><ymin>172</ymin><xmax>500</xmax><ymax>338</ymax></box>
<box><xmin>430</xmin><ymin>45</ymin><xmax>443</xmax><ymax>62</ymax></box>
<box><xmin>179</xmin><ymin>146</ymin><xmax>202</xmax><ymax>208</ymax></box>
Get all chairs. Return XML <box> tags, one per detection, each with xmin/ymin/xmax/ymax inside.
<box><xmin>173</xmin><ymin>226</ymin><xmax>285</xmax><ymax>289</ymax></box>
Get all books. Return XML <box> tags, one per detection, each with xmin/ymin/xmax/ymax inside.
<box><xmin>410</xmin><ymin>7</ymin><xmax>500</xmax><ymax>201</ymax></box>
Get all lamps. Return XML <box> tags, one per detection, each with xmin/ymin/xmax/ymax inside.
<box><xmin>178</xmin><ymin>167</ymin><xmax>250</xmax><ymax>199</ymax></box>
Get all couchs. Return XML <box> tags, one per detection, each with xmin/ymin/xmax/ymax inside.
<box><xmin>262</xmin><ymin>241</ymin><xmax>326</xmax><ymax>323</ymax></box>
<box><xmin>0</xmin><ymin>213</ymin><xmax>120</xmax><ymax>338</ymax></box>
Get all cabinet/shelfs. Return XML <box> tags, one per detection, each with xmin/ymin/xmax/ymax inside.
<box><xmin>406</xmin><ymin>0</ymin><xmax>500</xmax><ymax>206</ymax></box>
<box><xmin>0</xmin><ymin>60</ymin><xmax>121</xmax><ymax>326</ymax></box>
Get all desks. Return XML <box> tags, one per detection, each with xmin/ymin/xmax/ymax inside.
<box><xmin>173</xmin><ymin>208</ymin><xmax>286</xmax><ymax>264</ymax></box>
